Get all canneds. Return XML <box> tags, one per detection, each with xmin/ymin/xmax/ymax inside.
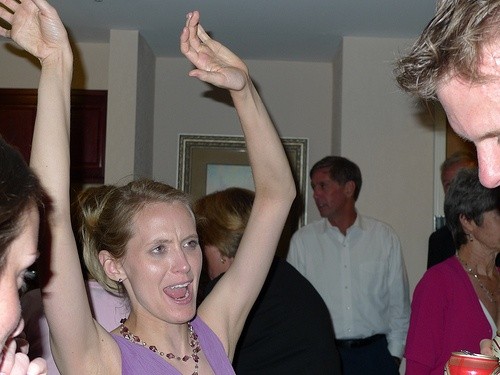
<box><xmin>443</xmin><ymin>349</ymin><xmax>500</xmax><ymax>375</ymax></box>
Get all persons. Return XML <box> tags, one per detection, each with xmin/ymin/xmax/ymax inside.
<box><xmin>391</xmin><ymin>0</ymin><xmax>500</xmax><ymax>375</ymax></box>
<box><xmin>0</xmin><ymin>1</ymin><xmax>337</xmax><ymax>374</ymax></box>
<box><xmin>287</xmin><ymin>156</ymin><xmax>411</xmax><ymax>375</ymax></box>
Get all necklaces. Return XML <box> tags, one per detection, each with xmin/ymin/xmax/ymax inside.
<box><xmin>120</xmin><ymin>317</ymin><xmax>201</xmax><ymax>375</ymax></box>
<box><xmin>455</xmin><ymin>251</ymin><xmax>498</xmax><ymax>303</ymax></box>
